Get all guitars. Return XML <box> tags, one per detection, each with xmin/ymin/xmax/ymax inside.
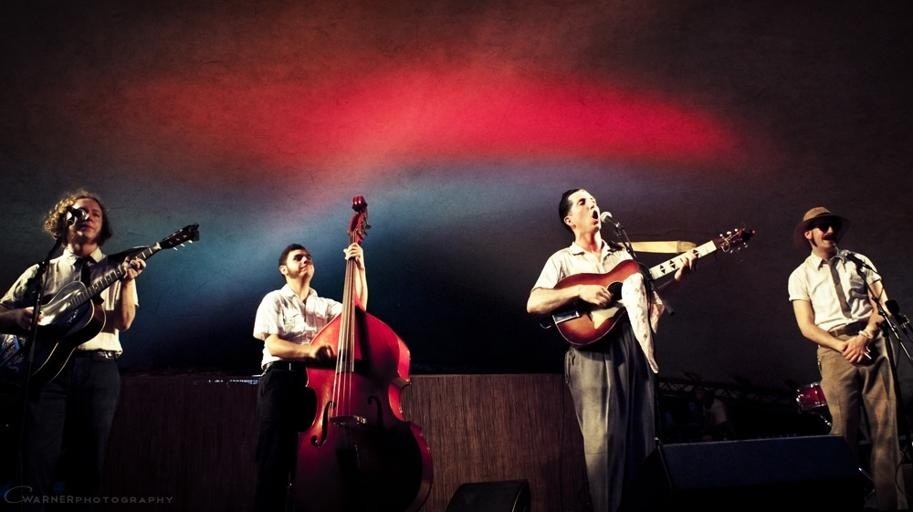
<box><xmin>549</xmin><ymin>224</ymin><xmax>757</xmax><ymax>350</ymax></box>
<box><xmin>0</xmin><ymin>223</ymin><xmax>198</xmax><ymax>391</ymax></box>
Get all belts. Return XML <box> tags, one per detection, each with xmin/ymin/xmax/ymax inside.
<box><xmin>826</xmin><ymin>315</ymin><xmax>873</xmax><ymax>339</ymax></box>
<box><xmin>92</xmin><ymin>349</ymin><xmax>118</xmax><ymax>361</ymax></box>
<box><xmin>261</xmin><ymin>361</ymin><xmax>306</xmax><ymax>371</ymax></box>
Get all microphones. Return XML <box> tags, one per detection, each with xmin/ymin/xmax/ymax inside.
<box><xmin>600</xmin><ymin>211</ymin><xmax>624</xmax><ymax>230</ymax></box>
<box><xmin>840</xmin><ymin>249</ymin><xmax>872</xmax><ymax>270</ymax></box>
<box><xmin>66</xmin><ymin>205</ymin><xmax>86</xmax><ymax>220</ymax></box>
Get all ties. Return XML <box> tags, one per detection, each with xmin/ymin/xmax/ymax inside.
<box><xmin>827</xmin><ymin>259</ymin><xmax>853</xmax><ymax>322</ymax></box>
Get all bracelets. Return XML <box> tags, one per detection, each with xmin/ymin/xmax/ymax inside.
<box><xmin>857</xmin><ymin>330</ymin><xmax>874</xmax><ymax>340</ymax></box>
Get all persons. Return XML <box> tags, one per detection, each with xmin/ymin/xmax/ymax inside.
<box><xmin>251</xmin><ymin>237</ymin><xmax>368</xmax><ymax>511</ymax></box>
<box><xmin>1</xmin><ymin>186</ymin><xmax>147</xmax><ymax>511</ymax></box>
<box><xmin>787</xmin><ymin>207</ymin><xmax>907</xmax><ymax>509</ymax></box>
<box><xmin>526</xmin><ymin>185</ymin><xmax>701</xmax><ymax>511</ymax></box>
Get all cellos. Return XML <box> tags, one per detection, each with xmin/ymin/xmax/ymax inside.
<box><xmin>296</xmin><ymin>197</ymin><xmax>433</xmax><ymax>512</ymax></box>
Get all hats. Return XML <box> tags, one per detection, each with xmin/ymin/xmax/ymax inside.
<box><xmin>792</xmin><ymin>205</ymin><xmax>852</xmax><ymax>250</ymax></box>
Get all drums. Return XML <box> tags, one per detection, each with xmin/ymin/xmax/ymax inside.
<box><xmin>795</xmin><ymin>383</ymin><xmax>826</xmax><ymax>415</ymax></box>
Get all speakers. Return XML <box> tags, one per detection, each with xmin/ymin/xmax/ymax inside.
<box><xmin>445</xmin><ymin>479</ymin><xmax>531</xmax><ymax>512</ymax></box>
<box><xmin>617</xmin><ymin>435</ymin><xmax>875</xmax><ymax>512</ymax></box>
